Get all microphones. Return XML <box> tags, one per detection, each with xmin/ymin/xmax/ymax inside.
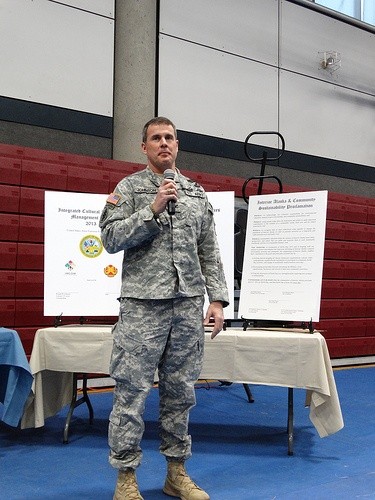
<box><xmin>164</xmin><ymin>169</ymin><xmax>176</xmax><ymax>215</ymax></box>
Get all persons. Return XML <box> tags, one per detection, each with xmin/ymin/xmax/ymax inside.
<box><xmin>100</xmin><ymin>116</ymin><xmax>229</xmax><ymax>499</ymax></box>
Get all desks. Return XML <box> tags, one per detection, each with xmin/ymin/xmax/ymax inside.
<box><xmin>16</xmin><ymin>324</ymin><xmax>344</xmax><ymax>457</ymax></box>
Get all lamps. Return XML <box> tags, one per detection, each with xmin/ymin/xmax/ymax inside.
<box><xmin>318</xmin><ymin>51</ymin><xmax>341</xmax><ymax>77</ymax></box>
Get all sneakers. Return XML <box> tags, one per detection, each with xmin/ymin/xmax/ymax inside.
<box><xmin>113</xmin><ymin>469</ymin><xmax>144</xmax><ymax>500</ymax></box>
<box><xmin>163</xmin><ymin>460</ymin><xmax>210</xmax><ymax>499</ymax></box>
